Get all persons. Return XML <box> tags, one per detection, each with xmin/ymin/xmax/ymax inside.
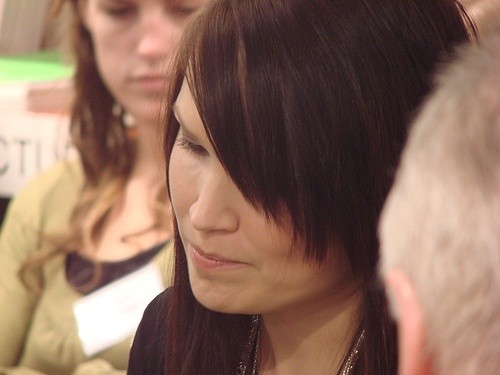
<box><xmin>123</xmin><ymin>0</ymin><xmax>484</xmax><ymax>375</ymax></box>
<box><xmin>1</xmin><ymin>0</ymin><xmax>203</xmax><ymax>375</ymax></box>
<box><xmin>378</xmin><ymin>31</ymin><xmax>499</xmax><ymax>375</ymax></box>
